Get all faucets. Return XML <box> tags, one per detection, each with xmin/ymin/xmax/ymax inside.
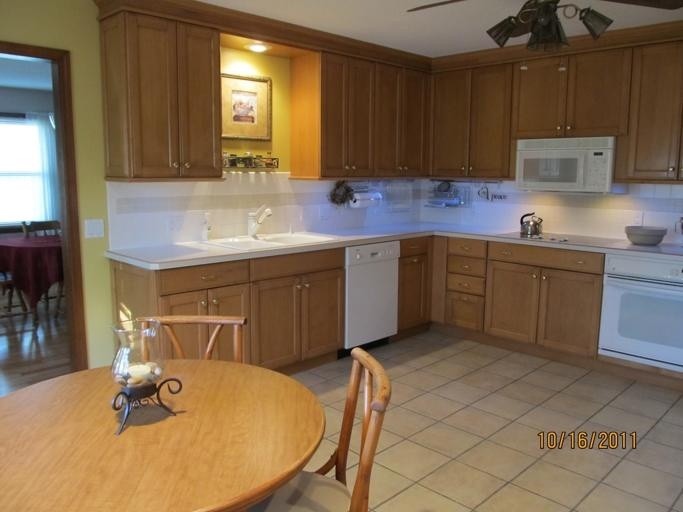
<box><xmin>247</xmin><ymin>208</ymin><xmax>272</xmax><ymax>237</ymax></box>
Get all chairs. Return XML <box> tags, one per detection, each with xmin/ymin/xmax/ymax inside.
<box><xmin>244</xmin><ymin>344</ymin><xmax>390</xmax><ymax>512</ymax></box>
<box><xmin>136</xmin><ymin>314</ymin><xmax>249</xmax><ymax>363</ymax></box>
<box><xmin>20</xmin><ymin>220</ymin><xmax>64</xmax><ymax>310</ymax></box>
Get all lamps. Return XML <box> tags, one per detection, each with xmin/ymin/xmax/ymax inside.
<box><xmin>486</xmin><ymin>0</ymin><xmax>614</xmax><ymax>51</ymax></box>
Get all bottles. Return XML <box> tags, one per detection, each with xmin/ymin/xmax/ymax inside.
<box><xmin>221</xmin><ymin>149</ymin><xmax>272</xmax><ymax>168</ymax></box>
<box><xmin>109</xmin><ymin>319</ymin><xmax>163</xmax><ymax>388</ymax></box>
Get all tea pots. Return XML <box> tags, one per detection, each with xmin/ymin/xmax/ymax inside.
<box><xmin>520</xmin><ymin>213</ymin><xmax>543</xmax><ymax>236</ymax></box>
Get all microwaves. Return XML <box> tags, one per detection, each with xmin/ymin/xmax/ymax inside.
<box><xmin>514</xmin><ymin>138</ymin><xmax>614</xmax><ymax>192</ymax></box>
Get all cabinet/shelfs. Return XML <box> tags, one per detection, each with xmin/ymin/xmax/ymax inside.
<box><xmin>485</xmin><ymin>235</ymin><xmax>605</xmax><ymax>369</ymax></box>
<box><xmin>431</xmin><ymin>63</ymin><xmax>513</xmax><ymax>184</ymax></box>
<box><xmin>250</xmin><ymin>246</ymin><xmax>345</xmax><ymax>379</ymax></box>
<box><xmin>512</xmin><ymin>46</ymin><xmax>634</xmax><ymax>138</ymax></box>
<box><xmin>108</xmin><ymin>259</ymin><xmax>250</xmax><ymax>373</ymax></box>
<box><xmin>392</xmin><ymin>233</ymin><xmax>432</xmax><ymax>342</ymax></box>
<box><xmin>627</xmin><ymin>42</ymin><xmax>680</xmax><ymax>185</ymax></box>
<box><xmin>433</xmin><ymin>233</ymin><xmax>488</xmax><ymax>344</ymax></box>
<box><xmin>95</xmin><ymin>2</ymin><xmax>225</xmax><ymax>183</ymax></box>
<box><xmin>289</xmin><ymin>52</ymin><xmax>373</xmax><ymax>180</ymax></box>
<box><xmin>373</xmin><ymin>63</ymin><xmax>428</xmax><ymax>178</ymax></box>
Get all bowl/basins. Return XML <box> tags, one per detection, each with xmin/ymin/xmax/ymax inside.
<box><xmin>624</xmin><ymin>223</ymin><xmax>667</xmax><ymax>246</ymax></box>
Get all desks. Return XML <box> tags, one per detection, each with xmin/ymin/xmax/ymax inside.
<box><xmin>0</xmin><ymin>238</ymin><xmax>61</xmax><ymax>323</ymax></box>
<box><xmin>0</xmin><ymin>358</ymin><xmax>326</xmax><ymax>512</ymax></box>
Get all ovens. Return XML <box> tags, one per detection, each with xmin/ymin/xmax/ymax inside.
<box><xmin>597</xmin><ymin>273</ymin><xmax>682</xmax><ymax>371</ymax></box>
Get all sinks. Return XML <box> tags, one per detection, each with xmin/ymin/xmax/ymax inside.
<box><xmin>263</xmin><ymin>233</ymin><xmax>332</xmax><ymax>246</ymax></box>
<box><xmin>216</xmin><ymin>239</ymin><xmax>284</xmax><ymax>251</ymax></box>
